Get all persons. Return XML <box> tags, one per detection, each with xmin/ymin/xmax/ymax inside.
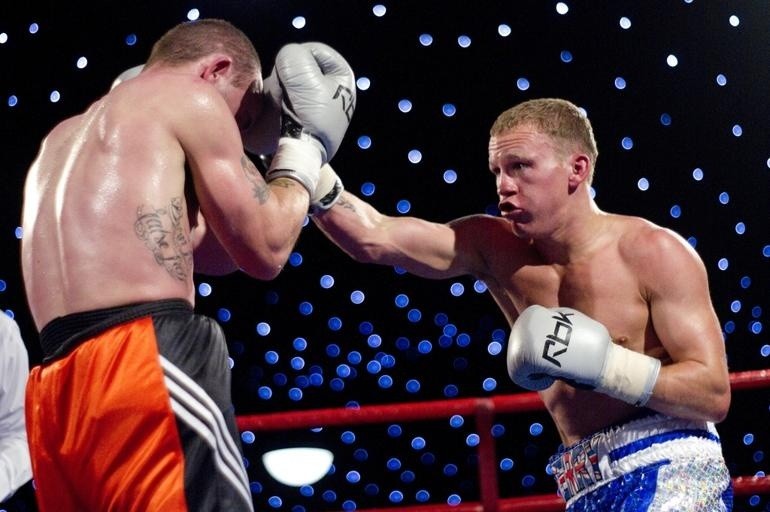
<box><xmin>243</xmin><ymin>98</ymin><xmax>732</xmax><ymax>511</ymax></box>
<box><xmin>22</xmin><ymin>21</ymin><xmax>356</xmax><ymax>511</ymax></box>
<box><xmin>1</xmin><ymin>312</ymin><xmax>39</xmax><ymax>512</ymax></box>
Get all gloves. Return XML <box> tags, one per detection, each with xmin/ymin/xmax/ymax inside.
<box><xmin>506</xmin><ymin>304</ymin><xmax>662</xmax><ymax>408</ymax></box>
<box><xmin>243</xmin><ymin>41</ymin><xmax>357</xmax><ymax>216</ymax></box>
